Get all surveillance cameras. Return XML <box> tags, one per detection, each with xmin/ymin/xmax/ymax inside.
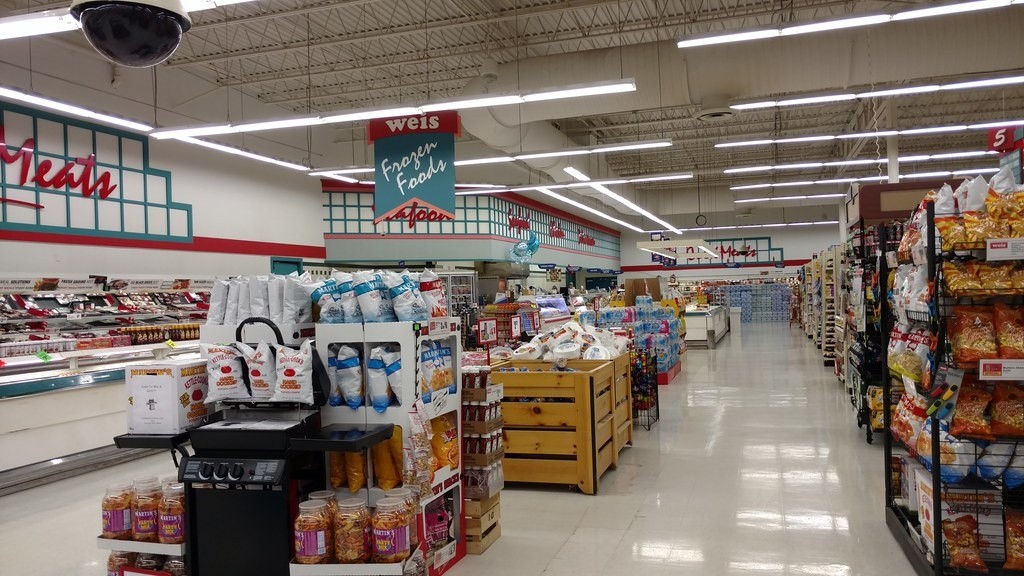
<box><xmin>69</xmin><ymin>0</ymin><xmax>192</xmax><ymax>67</ymax></box>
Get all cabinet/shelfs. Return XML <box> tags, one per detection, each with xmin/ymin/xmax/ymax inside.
<box><xmin>795</xmin><ymin>201</ymin><xmax>1023</xmax><ymax>575</ymax></box>
<box><xmin>0</xmin><ymin>281</ymin><xmax>731</xmax><ymax>576</ymax></box>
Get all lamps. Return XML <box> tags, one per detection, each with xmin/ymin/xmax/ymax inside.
<box><xmin>0</xmin><ymin>0</ymin><xmax>1023</xmax><ymax>260</ymax></box>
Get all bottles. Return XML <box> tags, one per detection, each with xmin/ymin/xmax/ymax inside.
<box><xmin>635</xmin><ymin>296</ymin><xmax>653</xmax><ymax>308</ymax></box>
<box><xmin>579</xmin><ymin>307</ymin><xmax>680</xmax><ymax>373</ymax></box>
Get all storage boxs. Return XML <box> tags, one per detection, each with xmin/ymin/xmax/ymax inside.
<box><xmin>124</xmin><ymin>358</ymin><xmax>215</xmax><ymax>434</ymax></box>
<box><xmin>720</xmin><ymin>284</ymin><xmax>792</xmax><ymax>323</ymax></box>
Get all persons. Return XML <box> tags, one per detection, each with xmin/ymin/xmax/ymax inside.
<box><xmin>568</xmin><ymin>282</ymin><xmax>575</xmax><ymax>295</ymax></box>
